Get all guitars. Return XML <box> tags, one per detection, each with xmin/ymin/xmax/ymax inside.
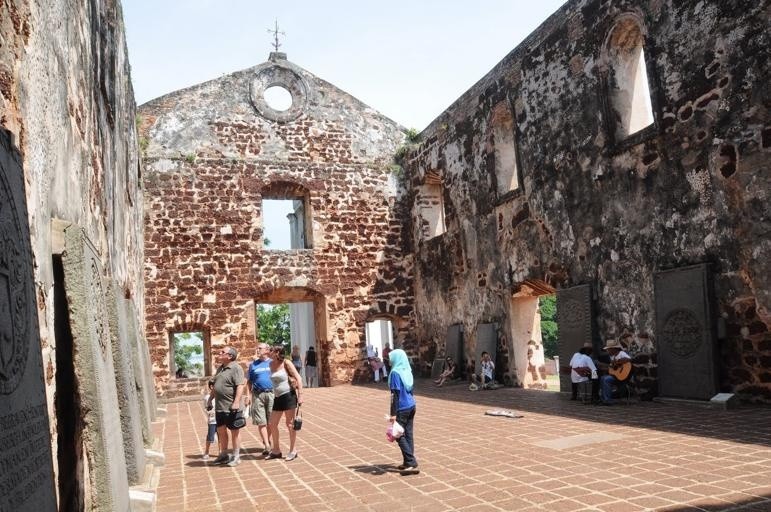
<box><xmin>593</xmin><ymin>359</ymin><xmax>632</xmax><ymax>381</ymax></box>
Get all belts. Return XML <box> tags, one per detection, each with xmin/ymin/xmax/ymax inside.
<box><xmin>254</xmin><ymin>388</ymin><xmax>272</xmax><ymax>392</ymax></box>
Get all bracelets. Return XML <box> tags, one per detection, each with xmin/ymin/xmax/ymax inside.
<box><xmin>299</xmin><ymin>392</ymin><xmax>303</xmax><ymax>394</ymax></box>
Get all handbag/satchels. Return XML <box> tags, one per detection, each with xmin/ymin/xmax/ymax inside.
<box><xmin>293</xmin><ymin>418</ymin><xmax>302</xmax><ymax>430</ymax></box>
<box><xmin>288</xmin><ymin>375</ymin><xmax>298</xmax><ymax>390</ymax></box>
<box><xmin>226</xmin><ymin>417</ymin><xmax>246</xmax><ymax>430</ymax></box>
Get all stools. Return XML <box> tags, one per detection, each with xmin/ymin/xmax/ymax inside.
<box><xmin>578</xmin><ymin>381</ymin><xmax>594</xmax><ymax>404</ymax></box>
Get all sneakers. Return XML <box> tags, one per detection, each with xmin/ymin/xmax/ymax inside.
<box><xmin>203</xmin><ymin>451</ymin><xmax>241</xmax><ymax>467</ymax></box>
<box><xmin>285</xmin><ymin>451</ymin><xmax>297</xmax><ymax>461</ymax></box>
<box><xmin>265</xmin><ymin>453</ymin><xmax>282</xmax><ymax>460</ymax></box>
<box><xmin>398</xmin><ymin>465</ymin><xmax>420</xmax><ymax>476</ymax></box>
<box><xmin>262</xmin><ymin>449</ymin><xmax>271</xmax><ymax>455</ymax></box>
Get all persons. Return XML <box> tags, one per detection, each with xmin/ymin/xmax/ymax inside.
<box><xmin>207</xmin><ymin>345</ymin><xmax>248</xmax><ymax>465</ymax></box>
<box><xmin>384</xmin><ymin>346</ymin><xmax>422</xmax><ymax>474</ymax></box>
<box><xmin>290</xmin><ymin>344</ymin><xmax>303</xmax><ymax>374</ymax></box>
<box><xmin>359</xmin><ymin>329</ymin><xmax>504</xmax><ymax>391</ymax></box>
<box><xmin>266</xmin><ymin>345</ymin><xmax>307</xmax><ymax>462</ymax></box>
<box><xmin>203</xmin><ymin>378</ymin><xmax>222</xmax><ymax>461</ymax></box>
<box><xmin>569</xmin><ymin>339</ymin><xmax>607</xmax><ymax>403</ymax></box>
<box><xmin>596</xmin><ymin>337</ymin><xmax>634</xmax><ymax>406</ymax></box>
<box><xmin>304</xmin><ymin>344</ymin><xmax>320</xmax><ymax>387</ymax></box>
<box><xmin>245</xmin><ymin>343</ymin><xmax>280</xmax><ymax>455</ymax></box>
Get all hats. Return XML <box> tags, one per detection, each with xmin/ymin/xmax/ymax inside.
<box><xmin>602</xmin><ymin>339</ymin><xmax>622</xmax><ymax>350</ymax></box>
<box><xmin>580</xmin><ymin>342</ymin><xmax>593</xmax><ymax>348</ymax></box>
